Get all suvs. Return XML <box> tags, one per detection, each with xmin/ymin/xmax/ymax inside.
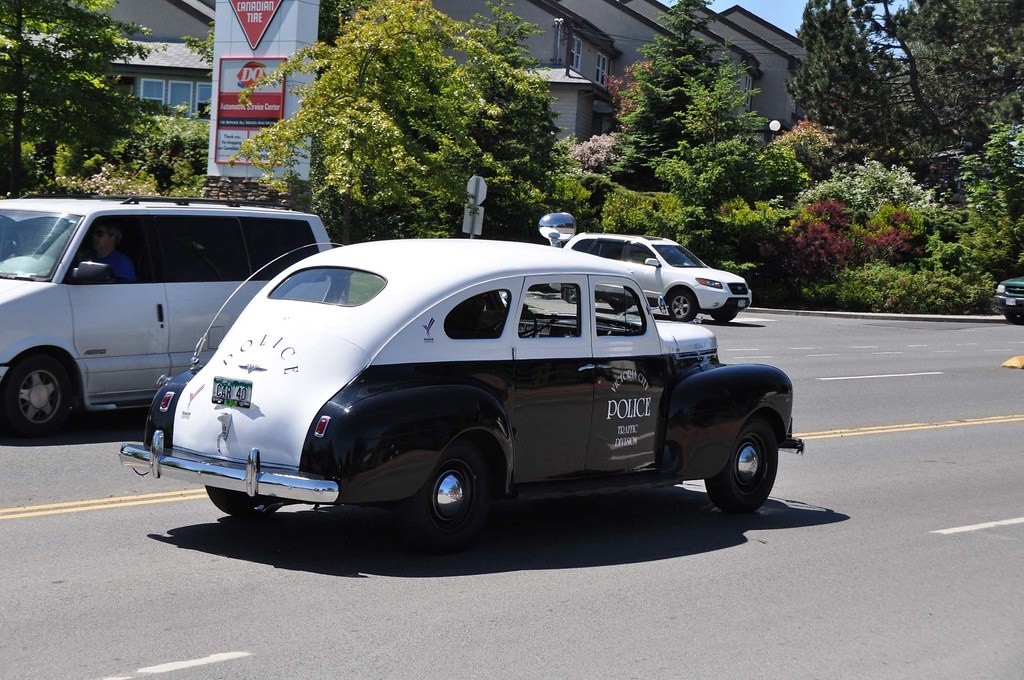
<box><xmin>549</xmin><ymin>230</ymin><xmax>754</xmax><ymax>324</ymax></box>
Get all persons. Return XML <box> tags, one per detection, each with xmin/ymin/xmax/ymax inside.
<box><xmin>79</xmin><ymin>221</ymin><xmax>137</xmax><ymax>281</ymax></box>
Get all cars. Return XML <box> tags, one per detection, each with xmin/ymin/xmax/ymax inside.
<box><xmin>992</xmin><ymin>276</ymin><xmax>1024</xmax><ymax>325</ymax></box>
<box><xmin>118</xmin><ymin>237</ymin><xmax>806</xmax><ymax>555</ymax></box>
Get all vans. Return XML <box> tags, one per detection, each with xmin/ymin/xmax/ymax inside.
<box><xmin>0</xmin><ymin>193</ymin><xmax>335</xmax><ymax>436</ymax></box>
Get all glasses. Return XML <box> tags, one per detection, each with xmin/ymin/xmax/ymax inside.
<box><xmin>90</xmin><ymin>230</ymin><xmax>108</xmax><ymax>237</ymax></box>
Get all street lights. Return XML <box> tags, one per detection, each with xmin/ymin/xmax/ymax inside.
<box><xmin>770</xmin><ymin>120</ymin><xmax>781</xmax><ymax>140</ymax></box>
